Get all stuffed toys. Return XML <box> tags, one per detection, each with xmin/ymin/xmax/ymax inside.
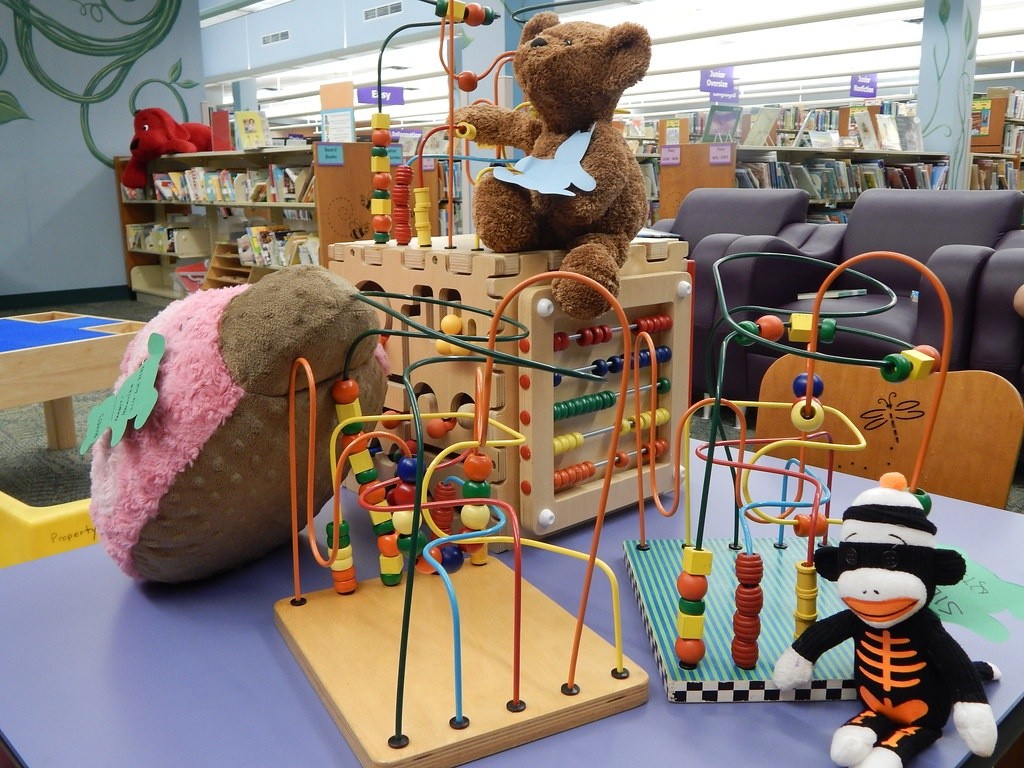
<box><xmin>773</xmin><ymin>471</ymin><xmax>998</xmax><ymax>768</ymax></box>
<box><xmin>79</xmin><ymin>261</ymin><xmax>392</xmax><ymax>585</ymax></box>
<box><xmin>445</xmin><ymin>13</ymin><xmax>651</xmax><ymax>318</ymax></box>
<box><xmin>122</xmin><ymin>108</ymin><xmax>213</xmax><ymax>187</ymax></box>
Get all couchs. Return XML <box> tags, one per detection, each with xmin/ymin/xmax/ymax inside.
<box><xmin>713</xmin><ymin>188</ymin><xmax>1024</xmax><ymax>430</ymax></box>
<box><xmin>969</xmin><ymin>229</ymin><xmax>1024</xmax><ymax>401</ymax></box>
<box><xmin>649</xmin><ymin>187</ymin><xmax>820</xmax><ymax>418</ymax></box>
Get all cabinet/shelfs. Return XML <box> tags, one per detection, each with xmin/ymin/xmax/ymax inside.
<box><xmin>114</xmin><ymin>141</ymin><xmax>374</xmax><ymax>305</ymax></box>
<box><xmin>201</xmin><ymin>242</ymin><xmax>278</xmax><ymax>291</ymax></box>
<box><xmin>435</xmin><ymin>86</ymin><xmax>1024</xmax><ymax>236</ymax></box>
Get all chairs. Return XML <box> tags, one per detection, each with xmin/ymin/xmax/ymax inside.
<box><xmin>754</xmin><ymin>351</ymin><xmax>1024</xmax><ymax>510</ymax></box>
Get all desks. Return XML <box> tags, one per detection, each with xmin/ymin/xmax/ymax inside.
<box><xmin>0</xmin><ymin>440</ymin><xmax>1023</xmax><ymax>768</ymax></box>
<box><xmin>0</xmin><ymin>311</ymin><xmax>148</xmax><ymax>450</ymax></box>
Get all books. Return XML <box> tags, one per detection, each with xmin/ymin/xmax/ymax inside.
<box><xmin>120</xmin><ymin>161</ymin><xmax>315</xmax><ymax>203</ymax></box>
<box><xmin>701</xmin><ymin>99</ymin><xmax>923</xmax><ymax>150</ymax></box>
<box><xmin>735</xmin><ymin>156</ymin><xmax>949</xmax><ymax>201</ymax></box>
<box><xmin>124</xmin><ymin>209</ymin><xmax>321</xmax><ymax>265</ymax></box>
<box><xmin>625</xmin><ymin>139</ymin><xmax>660</xmax><ymax>198</ymax></box>
<box><xmin>806</xmin><ymin>208</ymin><xmax>852</xmax><ymax>224</ymax></box>
<box><xmin>657</xmin><ymin>111</ymin><xmax>697</xmax><ymax>143</ymax></box>
<box><xmin>213</xmin><ymin>108</ymin><xmax>272</xmax><ymax>148</ymax></box>
<box><xmin>971</xmin><ymin>86</ymin><xmax>1023</xmax><ymax>190</ymax></box>
<box><xmin>394</xmin><ymin>125</ymin><xmax>459</xmax><ymax>156</ymax></box>
<box><xmin>438</xmin><ymin>159</ymin><xmax>462</xmax><ymax>235</ymax></box>
